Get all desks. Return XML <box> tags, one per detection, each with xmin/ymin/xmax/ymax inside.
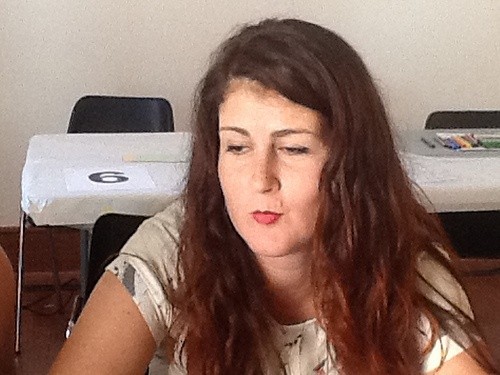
<box><xmin>15</xmin><ymin>128</ymin><xmax>500</xmax><ymax>336</ymax></box>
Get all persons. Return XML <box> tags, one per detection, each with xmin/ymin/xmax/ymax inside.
<box><xmin>1</xmin><ymin>246</ymin><xmax>18</xmax><ymax>373</ymax></box>
<box><xmin>46</xmin><ymin>19</ymin><xmax>500</xmax><ymax>375</ymax></box>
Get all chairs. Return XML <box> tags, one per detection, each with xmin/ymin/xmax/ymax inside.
<box><xmin>64</xmin><ymin>212</ymin><xmax>155</xmax><ymax>339</ymax></box>
<box><xmin>28</xmin><ymin>95</ymin><xmax>175</xmax><ymax>249</ymax></box>
<box><xmin>422</xmin><ymin>110</ymin><xmax>500</xmax><ymax>259</ymax></box>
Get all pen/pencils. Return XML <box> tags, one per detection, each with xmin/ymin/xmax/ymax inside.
<box><xmin>423</xmin><ymin>132</ymin><xmax>500</xmax><ymax>150</ymax></box>
